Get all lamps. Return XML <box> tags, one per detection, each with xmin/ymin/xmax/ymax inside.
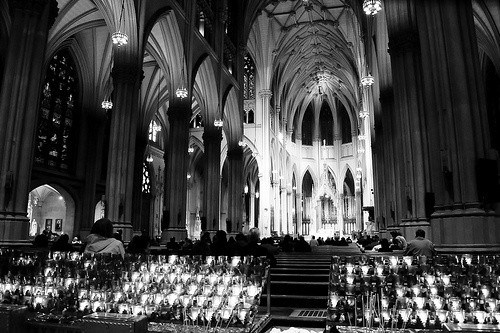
<box><xmin>363</xmin><ymin>0</ymin><xmax>384</xmax><ymax>16</ymax></box>
<box><xmin>354</xmin><ymin>73</ymin><xmax>375</xmax><ymax>190</ymax></box>
<box><xmin>87</xmin><ymin>0</ymin><xmax>223</xmax><ymax>180</ymax></box>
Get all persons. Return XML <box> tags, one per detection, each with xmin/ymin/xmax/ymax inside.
<box><xmin>404</xmin><ymin>229</ymin><xmax>436</xmax><ymax>259</ymax></box>
<box><xmin>32</xmin><ymin>230</ymin><xmax>48</xmax><ymax>248</ymax></box>
<box><xmin>189</xmin><ymin>230</ymin><xmax>311</xmax><ymax>256</ymax></box>
<box><xmin>358</xmin><ymin>234</ymin><xmax>393</xmax><ymax>252</ymax></box>
<box><xmin>80</xmin><ymin>218</ymin><xmax>126</xmax><ymax>259</ymax></box>
<box><xmin>127</xmin><ymin>234</ymin><xmax>149</xmax><ymax>253</ymax></box>
<box><xmin>310</xmin><ymin>235</ymin><xmax>352</xmax><ymax>247</ymax></box>
<box><xmin>52</xmin><ymin>234</ymin><xmax>75</xmax><ymax>252</ymax></box>
<box><xmin>390</xmin><ymin>231</ymin><xmax>407</xmax><ymax>250</ymax></box>
